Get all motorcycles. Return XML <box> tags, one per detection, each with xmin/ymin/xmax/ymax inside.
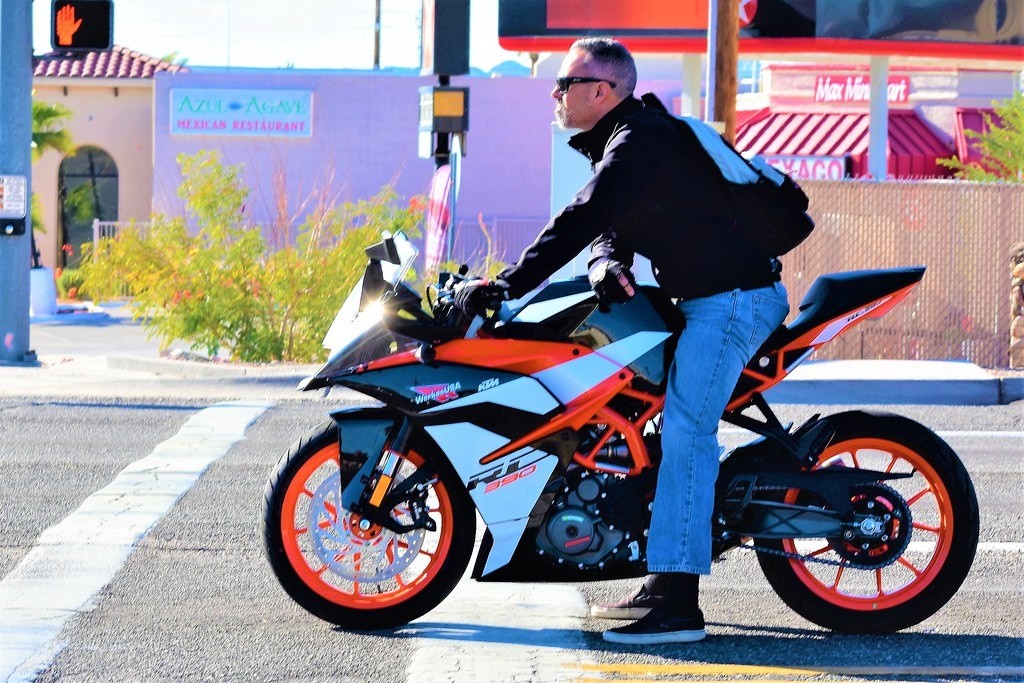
<box><xmin>257</xmin><ymin>227</ymin><xmax>979</xmax><ymax>637</ymax></box>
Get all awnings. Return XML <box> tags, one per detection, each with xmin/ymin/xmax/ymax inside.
<box><xmin>733</xmin><ymin>107</ymin><xmax>1017</xmax><ymax>180</ymax></box>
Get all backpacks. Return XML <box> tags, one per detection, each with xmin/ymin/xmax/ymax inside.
<box><xmin>600</xmin><ymin>91</ymin><xmax>815</xmax><ymax>259</ymax></box>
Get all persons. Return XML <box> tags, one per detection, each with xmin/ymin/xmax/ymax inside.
<box><xmin>454</xmin><ymin>37</ymin><xmax>790</xmax><ymax>645</ymax></box>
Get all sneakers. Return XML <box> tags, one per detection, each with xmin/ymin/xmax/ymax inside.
<box><xmin>602</xmin><ymin>604</ymin><xmax>705</xmax><ymax>643</ymax></box>
<box><xmin>590</xmin><ymin>580</ymin><xmax>664</xmax><ymax>619</ymax></box>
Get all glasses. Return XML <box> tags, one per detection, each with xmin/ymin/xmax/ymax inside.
<box><xmin>555</xmin><ymin>75</ymin><xmax>617</xmax><ymax>95</ymax></box>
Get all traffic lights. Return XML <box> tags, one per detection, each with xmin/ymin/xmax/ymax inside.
<box><xmin>49</xmin><ymin>1</ymin><xmax>114</xmax><ymax>53</ymax></box>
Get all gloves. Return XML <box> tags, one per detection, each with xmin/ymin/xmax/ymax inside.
<box><xmin>587</xmin><ymin>256</ymin><xmax>637</xmax><ymax>313</ymax></box>
<box><xmin>453</xmin><ymin>279</ymin><xmax>509</xmax><ymax>319</ymax></box>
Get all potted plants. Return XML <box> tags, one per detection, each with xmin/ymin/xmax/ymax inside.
<box><xmin>29</xmin><ymin>89</ymin><xmax>73</xmax><ymax>318</ymax></box>
